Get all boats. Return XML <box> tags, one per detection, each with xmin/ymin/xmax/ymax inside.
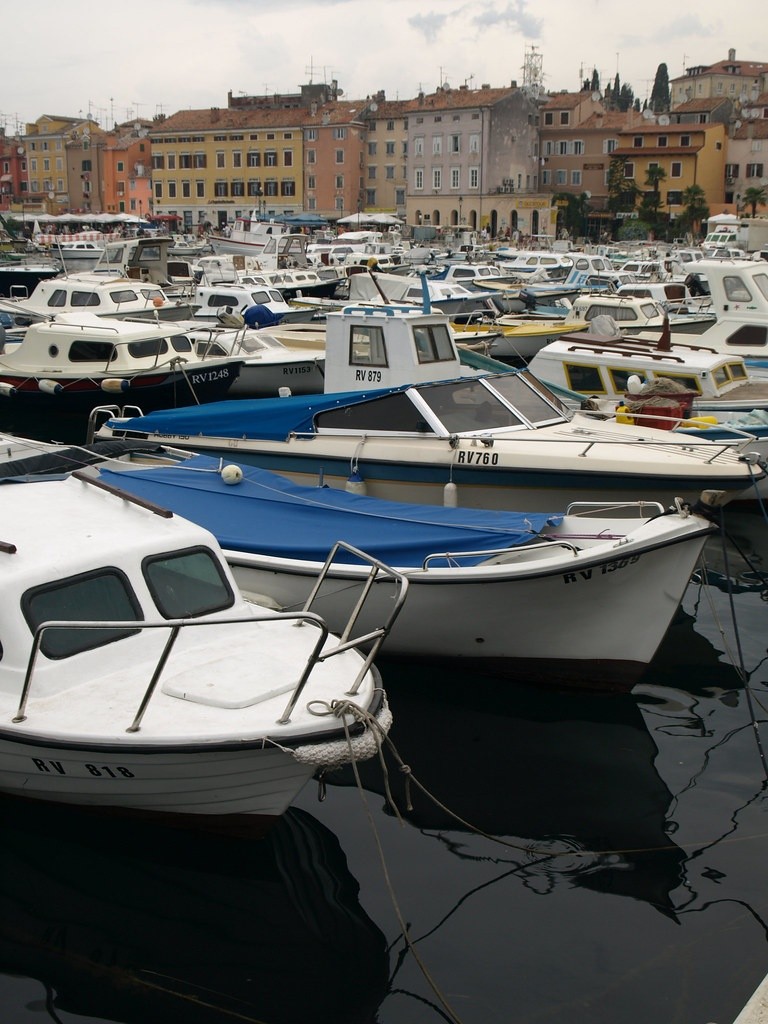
<box><xmin>0</xmin><ymin>219</ymin><xmax>767</xmax><ymax>518</ymax></box>
<box><xmin>63</xmin><ymin>444</ymin><xmax>717</xmax><ymax>694</ymax></box>
<box><xmin>0</xmin><ymin>472</ymin><xmax>410</xmax><ymax>822</ymax></box>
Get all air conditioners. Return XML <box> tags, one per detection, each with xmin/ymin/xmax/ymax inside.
<box><xmin>726</xmin><ymin>178</ymin><xmax>735</xmax><ymax>185</ymax></box>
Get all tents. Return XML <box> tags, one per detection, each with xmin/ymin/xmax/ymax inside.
<box><xmin>12</xmin><ymin>213</ymin><xmax>150</xmax><ymax>228</ymax></box>
<box><xmin>256</xmin><ymin>212</ymin><xmax>328</xmax><ymax>243</ymax></box>
<box><xmin>336</xmin><ymin>212</ymin><xmax>405</xmax><ymax>231</ymax></box>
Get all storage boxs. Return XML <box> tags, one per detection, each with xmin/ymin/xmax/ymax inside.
<box><xmin>635</xmin><ymin>400</ymin><xmax>688</xmax><ymax>430</ymax></box>
<box><xmin>624</xmin><ymin>391</ymin><xmax>702</xmax><ymax>419</ymax></box>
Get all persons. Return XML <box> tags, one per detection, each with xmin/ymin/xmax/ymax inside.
<box><xmin>45</xmin><ymin>222</ymin><xmax>230</xmax><ymax>238</ymax></box>
<box><xmin>481</xmin><ymin>223</ymin><xmax>608</xmax><ymax>251</ymax></box>
<box><xmin>299</xmin><ymin>224</ymin><xmax>398</xmax><ymax>236</ymax></box>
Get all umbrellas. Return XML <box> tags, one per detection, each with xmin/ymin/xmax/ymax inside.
<box><xmin>147</xmin><ymin>214</ymin><xmax>182</xmax><ymax>222</ymax></box>
